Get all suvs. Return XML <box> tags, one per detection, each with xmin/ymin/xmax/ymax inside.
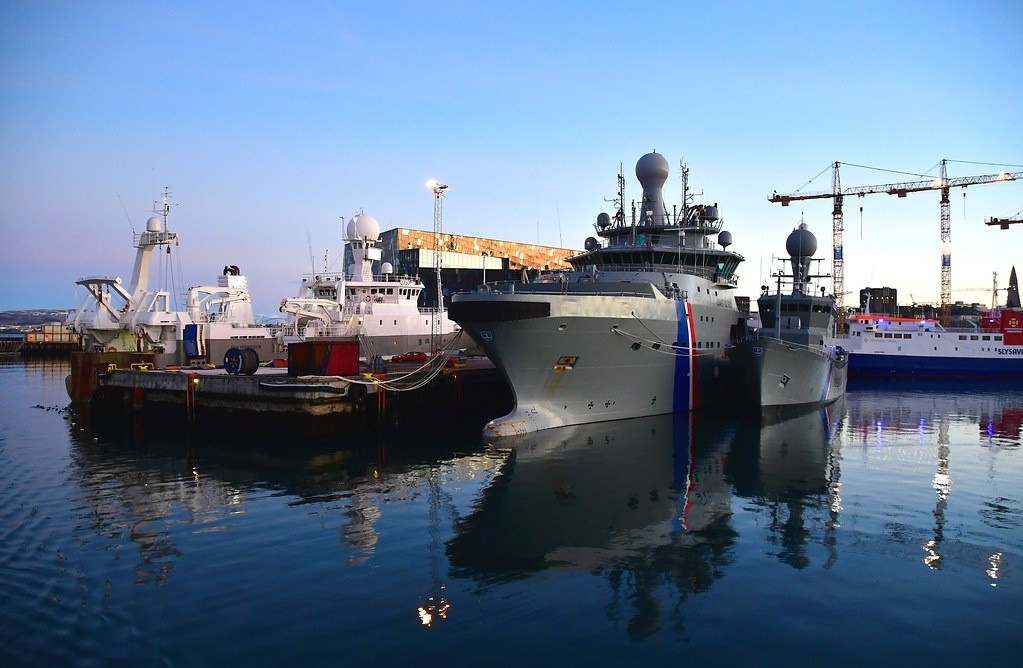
<box><xmin>392</xmin><ymin>350</ymin><xmax>425</xmax><ymax>362</ymax></box>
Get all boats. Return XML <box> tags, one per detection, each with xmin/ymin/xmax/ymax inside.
<box><xmin>442</xmin><ymin>150</ymin><xmax>752</xmax><ymax>439</ymax></box>
<box><xmin>836</xmin><ymin>311</ymin><xmax>1023</xmax><ymax>380</ymax></box>
<box><xmin>66</xmin><ymin>186</ymin><xmax>276</xmax><ymax>407</ymax></box>
<box><xmin>721</xmin><ymin>210</ymin><xmax>849</xmax><ymax>418</ymax></box>
<box><xmin>272</xmin><ymin>180</ymin><xmax>489</xmax><ymax>362</ymax></box>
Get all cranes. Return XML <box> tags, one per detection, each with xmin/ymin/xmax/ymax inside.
<box><xmin>766</xmin><ymin>160</ymin><xmax>967</xmax><ymax>337</ymax></box>
<box><xmin>983</xmin><ymin>209</ymin><xmax>1023</xmax><ymax>229</ymax></box>
<box><xmin>885</xmin><ymin>158</ymin><xmax>1023</xmax><ymax>326</ymax></box>
<box><xmin>950</xmin><ymin>271</ymin><xmax>1017</xmax><ymax>316</ymax></box>
